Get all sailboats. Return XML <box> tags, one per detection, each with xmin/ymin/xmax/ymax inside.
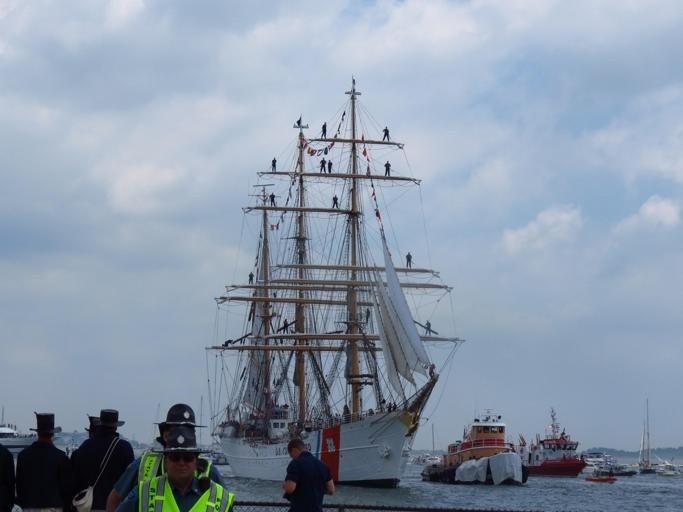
<box><xmin>203</xmin><ymin>78</ymin><xmax>468</xmax><ymax>493</ymax></box>
<box><xmin>140</xmin><ymin>392</ymin><xmax>227</xmax><ymax>466</ymax></box>
<box><xmin>635</xmin><ymin>397</ymin><xmax>656</xmax><ymax>476</ymax></box>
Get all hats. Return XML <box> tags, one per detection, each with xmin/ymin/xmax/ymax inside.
<box><xmin>93</xmin><ymin>408</ymin><xmax>125</xmax><ymax>428</ymax></box>
<box><xmin>151</xmin><ymin>427</ymin><xmax>212</xmax><ymax>457</ymax></box>
<box><xmin>152</xmin><ymin>402</ymin><xmax>208</xmax><ymax>430</ymax></box>
<box><xmin>28</xmin><ymin>412</ymin><xmax>62</xmax><ymax>435</ymax></box>
<box><xmin>84</xmin><ymin>416</ymin><xmax>101</xmax><ymax>432</ymax></box>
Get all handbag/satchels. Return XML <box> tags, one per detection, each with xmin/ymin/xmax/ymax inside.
<box><xmin>71</xmin><ymin>487</ymin><xmax>94</xmax><ymax>512</ymax></box>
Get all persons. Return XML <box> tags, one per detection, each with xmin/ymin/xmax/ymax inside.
<box><xmin>0</xmin><ymin>404</ymin><xmax>235</xmax><ymax>512</ymax></box>
<box><xmin>393</xmin><ymin>402</ymin><xmax>397</xmax><ymax>411</ymax></box>
<box><xmin>281</xmin><ymin>439</ymin><xmax>334</xmax><ymax>512</ymax></box>
<box><xmin>388</xmin><ymin>402</ymin><xmax>392</xmax><ymax>413</ymax></box>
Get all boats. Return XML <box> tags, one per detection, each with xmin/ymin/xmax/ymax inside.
<box><xmin>413</xmin><ymin>406</ymin><xmax>683</xmax><ymax>486</ymax></box>
<box><xmin>0</xmin><ymin>402</ymin><xmax>48</xmax><ymax>453</ymax></box>
